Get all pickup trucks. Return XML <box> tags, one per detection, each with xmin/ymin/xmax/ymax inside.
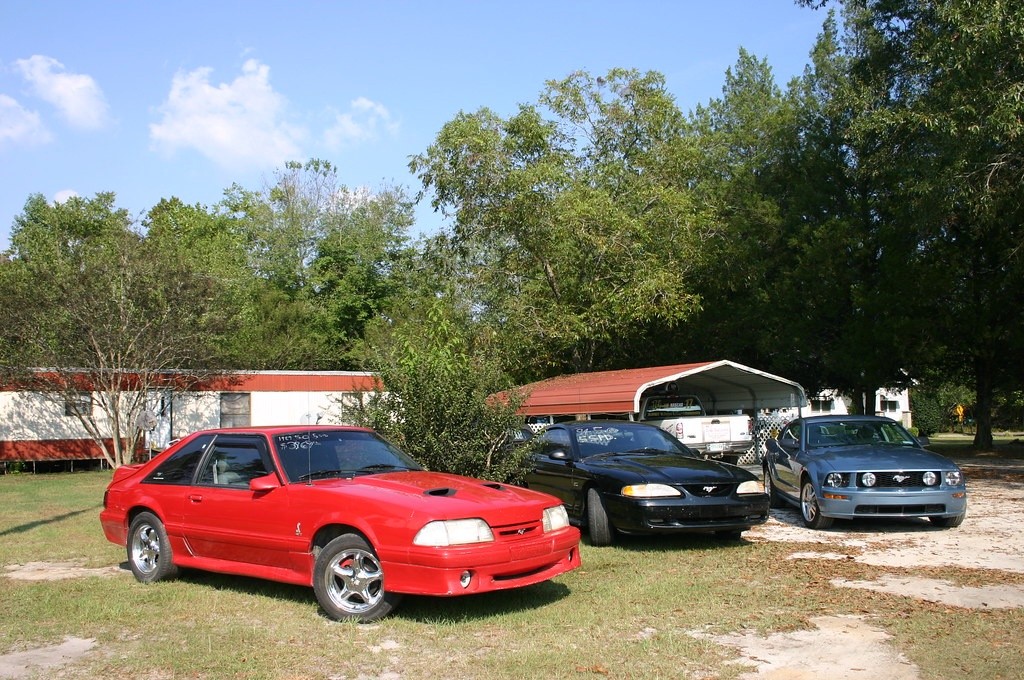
<box><xmin>636</xmin><ymin>394</ymin><xmax>754</xmax><ymax>466</ymax></box>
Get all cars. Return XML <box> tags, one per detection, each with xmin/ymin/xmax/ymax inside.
<box><xmin>99</xmin><ymin>424</ymin><xmax>581</xmax><ymax>622</ymax></box>
<box><xmin>506</xmin><ymin>424</ymin><xmax>535</xmax><ymax>451</ymax></box>
<box><xmin>522</xmin><ymin>420</ymin><xmax>770</xmax><ymax>547</ymax></box>
<box><xmin>764</xmin><ymin>414</ymin><xmax>968</xmax><ymax>528</ymax></box>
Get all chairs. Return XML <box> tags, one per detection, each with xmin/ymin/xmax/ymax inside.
<box><xmin>851</xmin><ymin>425</ymin><xmax>875</xmax><ymax>443</ymax></box>
<box><xmin>216</xmin><ymin>450</ymin><xmax>251</xmax><ymax>487</ymax></box>
